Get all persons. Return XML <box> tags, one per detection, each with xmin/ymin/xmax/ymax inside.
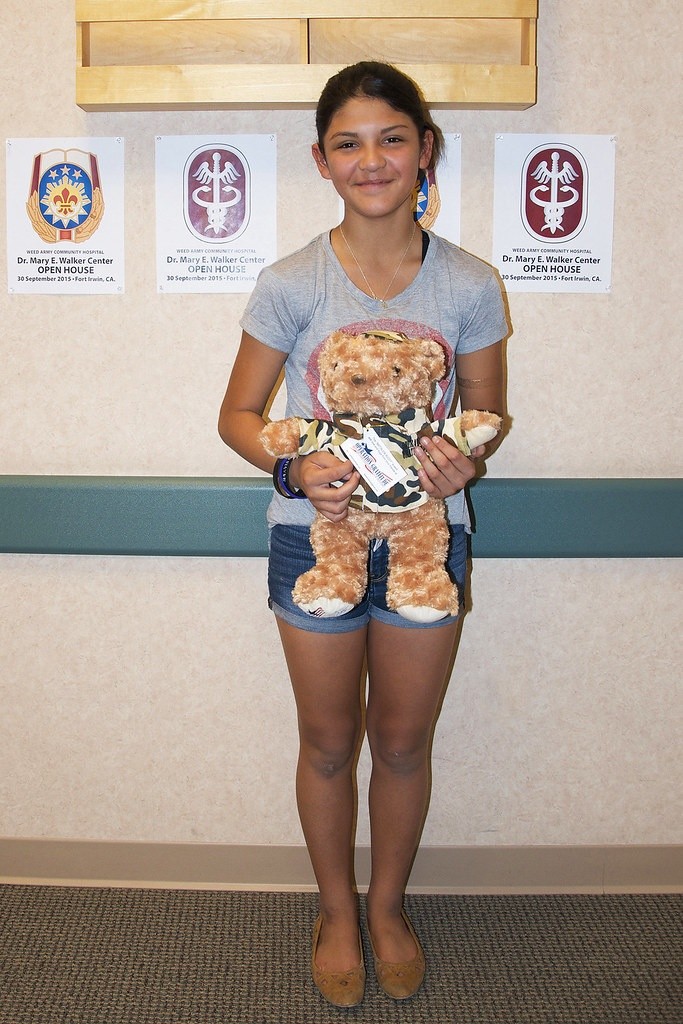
<box><xmin>218</xmin><ymin>61</ymin><xmax>510</xmax><ymax>1008</ymax></box>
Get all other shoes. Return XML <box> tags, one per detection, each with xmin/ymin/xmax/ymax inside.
<box><xmin>309</xmin><ymin>894</ymin><xmax>428</xmax><ymax>1005</ymax></box>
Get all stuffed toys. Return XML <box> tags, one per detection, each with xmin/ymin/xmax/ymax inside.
<box><xmin>259</xmin><ymin>329</ymin><xmax>501</xmax><ymax>623</ymax></box>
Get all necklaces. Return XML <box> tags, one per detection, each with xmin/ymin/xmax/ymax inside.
<box><xmin>340</xmin><ymin>224</ymin><xmax>415</xmax><ymax>307</ymax></box>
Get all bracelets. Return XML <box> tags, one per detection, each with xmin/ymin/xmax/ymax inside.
<box><xmin>273</xmin><ymin>457</ymin><xmax>307</xmax><ymax>500</ymax></box>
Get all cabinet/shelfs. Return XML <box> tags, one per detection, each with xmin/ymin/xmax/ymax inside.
<box><xmin>74</xmin><ymin>1</ymin><xmax>538</xmax><ymax>112</ymax></box>
